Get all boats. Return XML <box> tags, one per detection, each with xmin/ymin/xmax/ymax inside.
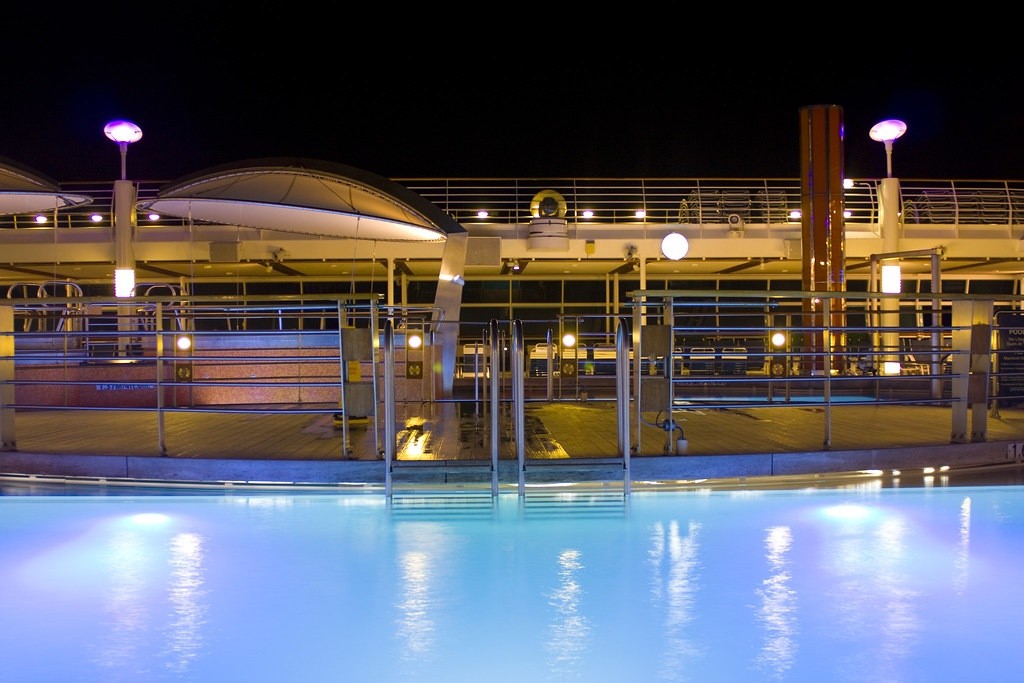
<box><xmin>0</xmin><ymin>106</ymin><xmax>1024</xmax><ymax>497</ymax></box>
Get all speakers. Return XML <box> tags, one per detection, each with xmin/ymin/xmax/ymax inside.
<box><xmin>208</xmin><ymin>241</ymin><xmax>242</xmax><ymax>263</ymax></box>
<box><xmin>784</xmin><ymin>238</ymin><xmax>802</xmax><ymax>259</ymax></box>
<box><xmin>465</xmin><ymin>237</ymin><xmax>501</xmax><ymax>266</ymax></box>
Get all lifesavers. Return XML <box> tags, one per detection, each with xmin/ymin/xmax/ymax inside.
<box><xmin>530</xmin><ymin>190</ymin><xmax>567</xmax><ymax>219</ymax></box>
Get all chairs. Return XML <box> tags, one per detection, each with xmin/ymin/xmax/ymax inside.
<box><xmin>677</xmin><ymin>187</ymin><xmax>789</xmax><ymax>224</ymax></box>
<box><xmin>901</xmin><ymin>187</ymin><xmax>1024</xmax><ymax>225</ymax></box>
<box><xmin>455</xmin><ymin>337</ymin><xmax>961</xmax><ymax>377</ymax></box>
